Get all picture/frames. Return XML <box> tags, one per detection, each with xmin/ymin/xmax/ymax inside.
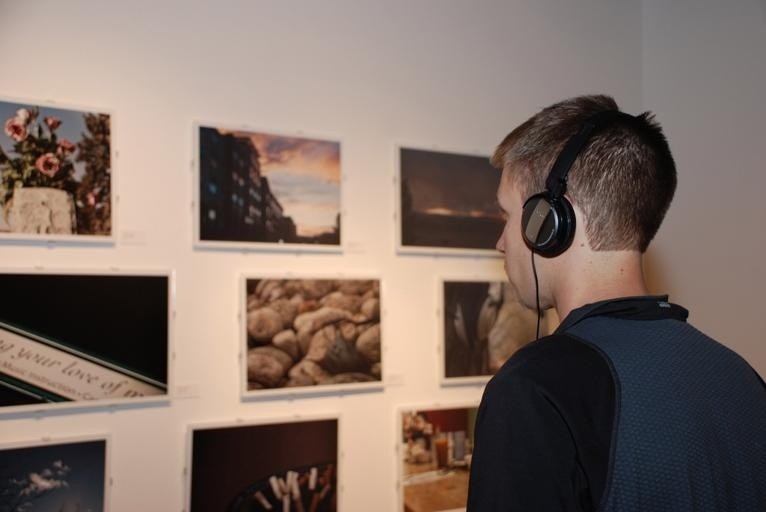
<box><xmin>0</xmin><ymin>95</ymin><xmax>115</xmax><ymax>244</ymax></box>
<box><xmin>192</xmin><ymin>121</ymin><xmax>345</xmax><ymax>253</ymax></box>
<box><xmin>0</xmin><ymin>433</ymin><xmax>110</xmax><ymax>512</ymax></box>
<box><xmin>394</xmin><ymin>144</ymin><xmax>502</xmax><ymax>257</ymax></box>
<box><xmin>437</xmin><ymin>275</ymin><xmax>559</xmax><ymax>386</ymax></box>
<box><xmin>185</xmin><ymin>413</ymin><xmax>344</xmax><ymax>512</ymax></box>
<box><xmin>397</xmin><ymin>400</ymin><xmax>478</xmax><ymax>512</ymax></box>
<box><xmin>0</xmin><ymin>267</ymin><xmax>174</xmax><ymax>414</ymax></box>
<box><xmin>238</xmin><ymin>273</ymin><xmax>387</xmax><ymax>400</ymax></box>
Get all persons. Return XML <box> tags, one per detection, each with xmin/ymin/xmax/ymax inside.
<box><xmin>468</xmin><ymin>95</ymin><xmax>766</xmax><ymax>512</ymax></box>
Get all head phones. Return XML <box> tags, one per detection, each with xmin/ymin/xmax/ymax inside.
<box><xmin>520</xmin><ymin>109</ymin><xmax>620</xmax><ymax>258</ymax></box>
